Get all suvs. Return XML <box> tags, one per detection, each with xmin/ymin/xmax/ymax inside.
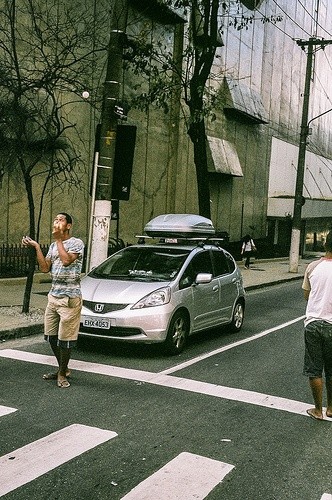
<box><xmin>79</xmin><ymin>214</ymin><xmax>246</xmax><ymax>356</ymax></box>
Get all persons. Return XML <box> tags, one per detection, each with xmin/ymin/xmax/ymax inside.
<box><xmin>301</xmin><ymin>230</ymin><xmax>332</xmax><ymax>419</ymax></box>
<box><xmin>22</xmin><ymin>213</ymin><xmax>84</xmax><ymax>387</ymax></box>
<box><xmin>241</xmin><ymin>234</ymin><xmax>257</xmax><ymax>270</ymax></box>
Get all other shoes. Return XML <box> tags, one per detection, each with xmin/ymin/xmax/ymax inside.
<box><xmin>244</xmin><ymin>266</ymin><xmax>249</xmax><ymax>269</ymax></box>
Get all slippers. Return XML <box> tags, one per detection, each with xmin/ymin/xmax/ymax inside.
<box><xmin>43</xmin><ymin>371</ymin><xmax>58</xmax><ymax>379</ymax></box>
<box><xmin>57</xmin><ymin>376</ymin><xmax>70</xmax><ymax>388</ymax></box>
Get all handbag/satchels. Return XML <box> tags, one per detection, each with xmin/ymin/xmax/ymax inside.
<box><xmin>250</xmin><ymin>240</ymin><xmax>257</xmax><ymax>251</ymax></box>
